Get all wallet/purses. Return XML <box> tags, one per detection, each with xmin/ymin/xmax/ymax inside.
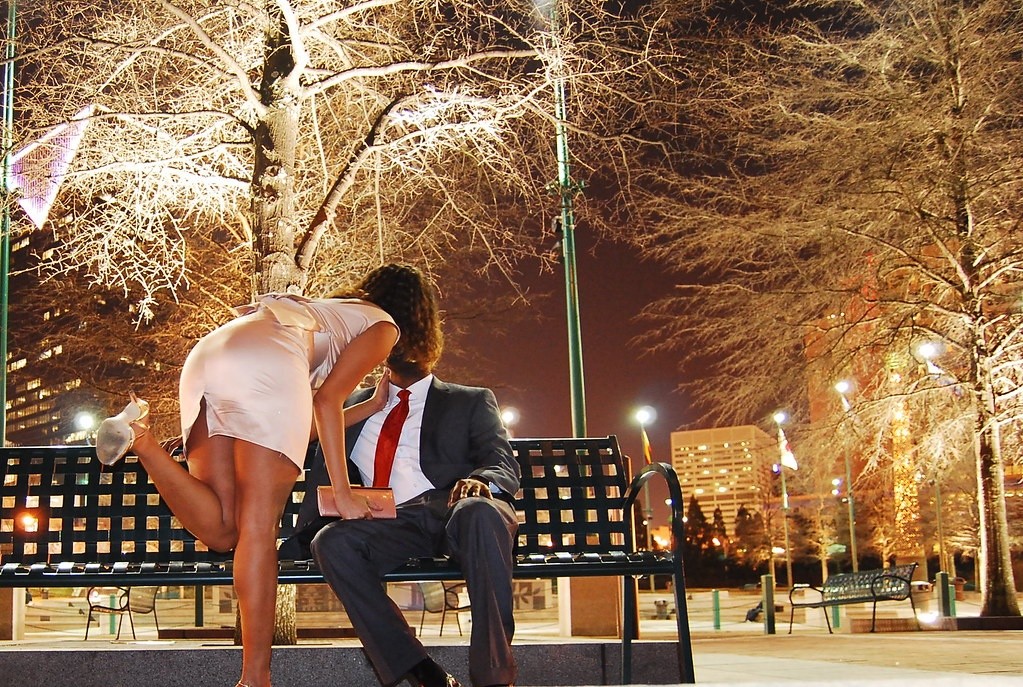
<box><xmin>317</xmin><ymin>484</ymin><xmax>398</xmax><ymax>519</ymax></box>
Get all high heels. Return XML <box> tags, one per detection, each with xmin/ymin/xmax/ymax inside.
<box><xmin>95</xmin><ymin>391</ymin><xmax>150</xmax><ymax>465</ymax></box>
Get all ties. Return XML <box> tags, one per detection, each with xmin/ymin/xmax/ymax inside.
<box><xmin>373</xmin><ymin>389</ymin><xmax>411</xmax><ymax>488</ymax></box>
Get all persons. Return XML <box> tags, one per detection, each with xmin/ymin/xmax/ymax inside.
<box><xmin>97</xmin><ymin>260</ymin><xmax>444</xmax><ymax>687</ymax></box>
<box><xmin>276</xmin><ymin>330</ymin><xmax>522</xmax><ymax>687</ymax></box>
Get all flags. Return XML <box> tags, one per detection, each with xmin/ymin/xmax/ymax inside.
<box><xmin>644</xmin><ymin>428</ymin><xmax>652</xmax><ymax>464</ymax></box>
<box><xmin>780</xmin><ymin>429</ymin><xmax>798</xmax><ymax>471</ymax></box>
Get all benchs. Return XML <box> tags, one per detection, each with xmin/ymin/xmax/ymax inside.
<box><xmin>414</xmin><ymin>580</ymin><xmax>472</xmax><ymax>638</ymax></box>
<box><xmin>0</xmin><ymin>432</ymin><xmax>697</xmax><ymax>687</ymax></box>
<box><xmin>80</xmin><ymin>584</ymin><xmax>159</xmax><ymax>641</ymax></box>
<box><xmin>790</xmin><ymin>562</ymin><xmax>922</xmax><ymax>634</ymax></box>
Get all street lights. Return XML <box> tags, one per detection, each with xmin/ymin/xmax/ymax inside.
<box><xmin>636</xmin><ymin>409</ymin><xmax>656</xmax><ymax>593</ymax></box>
<box><xmin>79</xmin><ymin>415</ymin><xmax>91</xmax><ymax>506</ymax></box>
<box><xmin>918</xmin><ymin>344</ymin><xmax>950</xmax><ymax>571</ymax></box>
<box><xmin>502</xmin><ymin>407</ymin><xmax>518</xmax><ymax>444</ymax></box>
<box><xmin>774</xmin><ymin>413</ymin><xmax>794</xmax><ymax>589</ymax></box>
<box><xmin>836</xmin><ymin>381</ymin><xmax>859</xmax><ymax>589</ymax></box>
